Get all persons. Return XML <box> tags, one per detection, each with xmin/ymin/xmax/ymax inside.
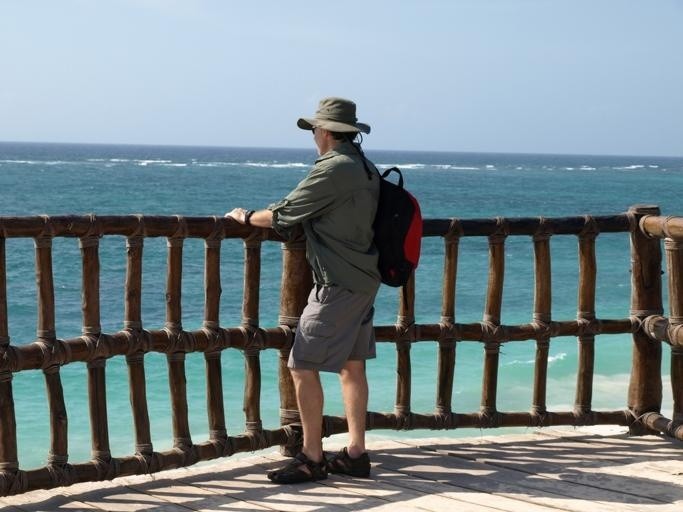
<box><xmin>222</xmin><ymin>96</ymin><xmax>383</xmax><ymax>485</ymax></box>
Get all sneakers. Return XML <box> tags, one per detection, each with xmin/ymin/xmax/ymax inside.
<box><xmin>323</xmin><ymin>447</ymin><xmax>371</xmax><ymax>478</ymax></box>
<box><xmin>267</xmin><ymin>453</ymin><xmax>329</xmax><ymax>485</ymax></box>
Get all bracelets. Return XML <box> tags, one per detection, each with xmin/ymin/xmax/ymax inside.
<box><xmin>243</xmin><ymin>209</ymin><xmax>255</xmax><ymax>228</ymax></box>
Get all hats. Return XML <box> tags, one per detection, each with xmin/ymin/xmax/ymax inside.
<box><xmin>295</xmin><ymin>97</ymin><xmax>371</xmax><ymax>137</ymax></box>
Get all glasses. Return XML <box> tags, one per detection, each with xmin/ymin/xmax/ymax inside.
<box><xmin>311</xmin><ymin>125</ymin><xmax>318</xmax><ymax>136</ymax></box>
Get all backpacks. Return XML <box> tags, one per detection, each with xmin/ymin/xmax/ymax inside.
<box><xmin>368</xmin><ymin>158</ymin><xmax>423</xmax><ymax>290</ymax></box>
